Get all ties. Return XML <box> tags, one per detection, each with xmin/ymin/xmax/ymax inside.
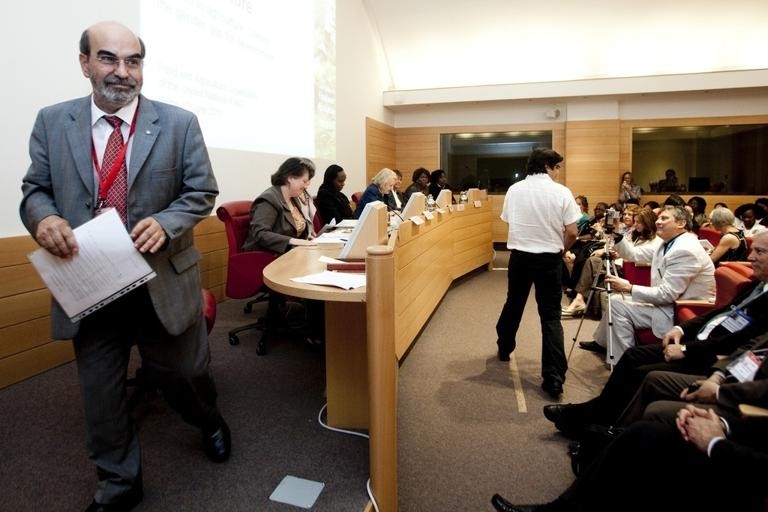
<box><xmin>97</xmin><ymin>116</ymin><xmax>128</xmax><ymax>232</ymax></box>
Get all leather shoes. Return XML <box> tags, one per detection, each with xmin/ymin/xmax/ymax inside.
<box><xmin>491</xmin><ymin>493</ymin><xmax>514</xmax><ymax>508</ymax></box>
<box><xmin>545</xmin><ymin>403</ymin><xmax>572</xmax><ymax>430</ymax></box>
<box><xmin>199</xmin><ymin>409</ymin><xmax>231</xmax><ymax>463</ymax></box>
<box><xmin>543</xmin><ymin>380</ymin><xmax>562</xmax><ymax>399</ymax></box>
<box><xmin>581</xmin><ymin>341</ymin><xmax>607</xmax><ymax>354</ymax></box>
<box><xmin>497</xmin><ymin>349</ymin><xmax>511</xmax><ymax>362</ymax></box>
<box><xmin>85</xmin><ymin>492</ymin><xmax>142</xmax><ymax>508</ymax></box>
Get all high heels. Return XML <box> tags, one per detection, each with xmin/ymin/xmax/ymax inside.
<box><xmin>561</xmin><ymin>305</ymin><xmax>585</xmax><ymax>316</ymax></box>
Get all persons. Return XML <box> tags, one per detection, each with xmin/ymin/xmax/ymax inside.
<box><xmin>574</xmin><ymin>203</ymin><xmax>610</xmax><ymax>279</ymax></box>
<box><xmin>659</xmin><ymin>171</ymin><xmax>676</xmax><ymax>192</ymax></box>
<box><xmin>489</xmin><ymin>403</ymin><xmax>768</xmax><ymax>511</ymax></box>
<box><xmin>687</xmin><ymin>197</ymin><xmax>708</xmax><ymax>229</ymax></box>
<box><xmin>358</xmin><ymin>168</ymin><xmax>396</xmax><ymax>222</ymax></box>
<box><xmin>379</xmin><ymin>169</ymin><xmax>406</xmax><ymax>213</ymax></box>
<box><xmin>317</xmin><ymin>165</ymin><xmax>354</xmax><ymax>225</ymax></box>
<box><xmin>581</xmin><ymin>207</ymin><xmax>715</xmax><ymax>373</ymax></box>
<box><xmin>622</xmin><ymin>204</ymin><xmax>642</xmax><ymax>227</ymax></box>
<box><xmin>618</xmin><ymin>173</ymin><xmax>643</xmax><ymax>203</ymax></box>
<box><xmin>406</xmin><ymin>167</ymin><xmax>432</xmax><ymax>211</ymax></box>
<box><xmin>692</xmin><ymin>211</ymin><xmax>749</xmax><ymax>263</ymax></box>
<box><xmin>546</xmin><ymin>232</ymin><xmax>767</xmax><ymax>443</ymax></box>
<box><xmin>564</xmin><ymin>196</ymin><xmax>595</xmax><ymax>291</ymax></box>
<box><xmin>21</xmin><ymin>19</ymin><xmax>233</xmax><ymax>511</ymax></box>
<box><xmin>242</xmin><ymin>157</ymin><xmax>321</xmax><ymax>254</ymax></box>
<box><xmin>566</xmin><ymin>332</ymin><xmax>768</xmax><ymax>457</ymax></box>
<box><xmin>496</xmin><ymin>148</ymin><xmax>581</xmax><ymax>397</ymax></box>
<box><xmin>561</xmin><ymin>208</ymin><xmax>665</xmax><ymax>316</ymax></box>
<box><xmin>429</xmin><ymin>170</ymin><xmax>456</xmax><ymax>207</ymax></box>
<box><xmin>736</xmin><ymin>204</ymin><xmax>764</xmax><ymax>235</ymax></box>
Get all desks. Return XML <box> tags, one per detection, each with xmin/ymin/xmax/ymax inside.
<box><xmin>261</xmin><ymin>197</ymin><xmax>492</xmax><ymax>512</ymax></box>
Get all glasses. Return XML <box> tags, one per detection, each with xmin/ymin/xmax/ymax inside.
<box><xmin>82</xmin><ymin>53</ymin><xmax>144</xmax><ymax>68</ymax></box>
<box><xmin>552</xmin><ymin>162</ymin><xmax>561</xmax><ymax>169</ymax></box>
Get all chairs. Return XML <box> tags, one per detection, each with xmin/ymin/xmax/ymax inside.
<box><xmin>351</xmin><ymin>192</ymin><xmax>363</xmax><ymax>204</ymax></box>
<box><xmin>635</xmin><ymin>328</ymin><xmax>661</xmax><ymax>345</ymax></box>
<box><xmin>216</xmin><ymin>199</ymin><xmax>295</xmax><ymax>356</ymax></box>
<box><xmin>725</xmin><ymin>262</ymin><xmax>754</xmax><ymax>278</ymax></box>
<box><xmin>716</xmin><ymin>353</ymin><xmax>768</xmax><ymax>420</ymax></box>
<box><xmin>124</xmin><ymin>287</ymin><xmax>217</xmax><ymax>420</ymax></box>
<box><xmin>623</xmin><ymin>259</ymin><xmax>650</xmax><ymax>288</ymax></box>
<box><xmin>311</xmin><ymin>196</ymin><xmax>322</xmax><ymax>234</ymax></box>
<box><xmin>699</xmin><ymin>226</ymin><xmax>721</xmax><ymax>249</ymax></box>
<box><xmin>676</xmin><ymin>266</ymin><xmax>752</xmax><ymax>323</ymax></box>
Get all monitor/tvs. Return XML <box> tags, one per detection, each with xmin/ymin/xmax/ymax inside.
<box><xmin>341</xmin><ymin>200</ymin><xmax>390</xmax><ymax>261</ymax></box>
<box><xmin>396</xmin><ymin>193</ymin><xmax>425</xmax><ymax>228</ymax></box>
<box><xmin>468</xmin><ymin>188</ymin><xmax>488</xmax><ymax>202</ymax></box>
<box><xmin>434</xmin><ymin>190</ymin><xmax>452</xmax><ymax>207</ymax></box>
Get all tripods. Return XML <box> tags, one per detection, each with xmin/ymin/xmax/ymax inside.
<box><xmin>568</xmin><ymin>235</ymin><xmax>638</xmax><ymax>374</ymax></box>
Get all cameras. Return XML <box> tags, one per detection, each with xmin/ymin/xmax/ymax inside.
<box><xmin>603</xmin><ymin>209</ymin><xmax>620</xmax><ymax>229</ymax></box>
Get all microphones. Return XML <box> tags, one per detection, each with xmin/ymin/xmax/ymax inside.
<box><xmin>377</xmin><ymin>198</ymin><xmax>404</xmax><ymax>223</ymax></box>
